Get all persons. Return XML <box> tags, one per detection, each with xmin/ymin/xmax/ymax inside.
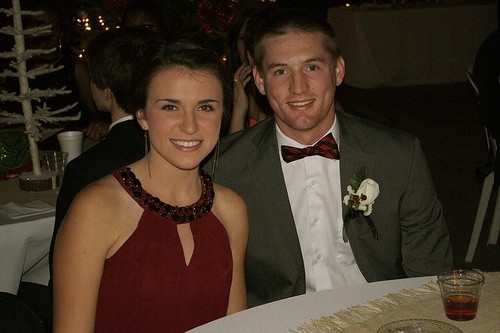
<box><xmin>15</xmin><ymin>0</ymin><xmax>276</xmax><ymax>234</ymax></box>
<box><xmin>201</xmin><ymin>7</ymin><xmax>453</xmax><ymax>311</ymax></box>
<box><xmin>50</xmin><ymin>43</ymin><xmax>248</xmax><ymax>333</ymax></box>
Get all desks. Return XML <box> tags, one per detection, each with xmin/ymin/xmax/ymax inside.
<box><xmin>185</xmin><ymin>271</ymin><xmax>500</xmax><ymax>333</ymax></box>
<box><xmin>0</xmin><ymin>149</ymin><xmax>60</xmax><ymax>295</ymax></box>
<box><xmin>327</xmin><ymin>0</ymin><xmax>498</xmax><ymax>89</ymax></box>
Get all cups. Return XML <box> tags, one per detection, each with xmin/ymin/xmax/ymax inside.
<box><xmin>43</xmin><ymin>151</ymin><xmax>69</xmax><ymax>170</ymax></box>
<box><xmin>57</xmin><ymin>131</ymin><xmax>83</xmax><ymax>163</ymax></box>
<box><xmin>437</xmin><ymin>269</ymin><xmax>485</xmax><ymax>321</ymax></box>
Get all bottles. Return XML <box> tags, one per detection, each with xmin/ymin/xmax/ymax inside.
<box><xmin>0</xmin><ymin>142</ymin><xmax>18</xmax><ymax>179</ymax></box>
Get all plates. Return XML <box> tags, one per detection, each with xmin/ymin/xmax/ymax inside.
<box><xmin>376</xmin><ymin>319</ymin><xmax>463</xmax><ymax>333</ymax></box>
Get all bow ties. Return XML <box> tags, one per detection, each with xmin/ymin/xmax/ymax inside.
<box><xmin>281</xmin><ymin>132</ymin><xmax>341</xmax><ymax>163</ymax></box>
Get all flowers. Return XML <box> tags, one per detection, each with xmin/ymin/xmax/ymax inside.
<box><xmin>342</xmin><ymin>165</ymin><xmax>380</xmax><ymax>243</ymax></box>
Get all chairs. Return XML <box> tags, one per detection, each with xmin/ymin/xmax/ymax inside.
<box><xmin>465</xmin><ymin>73</ymin><xmax>500</xmax><ymax>262</ymax></box>
